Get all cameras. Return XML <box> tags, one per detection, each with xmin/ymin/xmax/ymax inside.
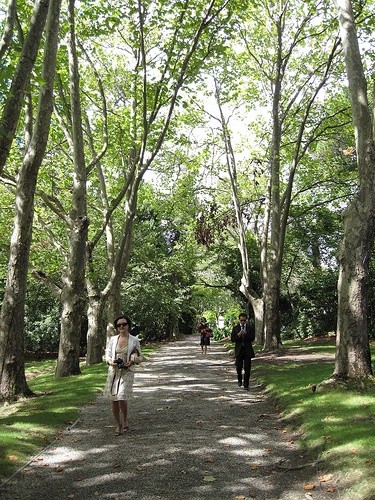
<box><xmin>114</xmin><ymin>358</ymin><xmax>124</xmax><ymax>369</ymax></box>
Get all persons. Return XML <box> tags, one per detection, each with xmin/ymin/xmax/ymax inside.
<box><xmin>197</xmin><ymin>317</ymin><xmax>210</xmax><ymax>354</ymax></box>
<box><xmin>231</xmin><ymin>313</ymin><xmax>255</xmax><ymax>390</ymax></box>
<box><xmin>104</xmin><ymin>316</ymin><xmax>141</xmax><ymax>435</ymax></box>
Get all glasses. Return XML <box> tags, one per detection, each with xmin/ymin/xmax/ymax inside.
<box><xmin>240</xmin><ymin>319</ymin><xmax>246</xmax><ymax>322</ymax></box>
<box><xmin>117</xmin><ymin>323</ymin><xmax>127</xmax><ymax>327</ymax></box>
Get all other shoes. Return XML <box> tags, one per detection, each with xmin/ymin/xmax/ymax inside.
<box><xmin>244</xmin><ymin>388</ymin><xmax>250</xmax><ymax>390</ymax></box>
<box><xmin>238</xmin><ymin>380</ymin><xmax>242</xmax><ymax>387</ymax></box>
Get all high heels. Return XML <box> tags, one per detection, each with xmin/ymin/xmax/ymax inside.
<box><xmin>114</xmin><ymin>424</ymin><xmax>122</xmax><ymax>435</ymax></box>
<box><xmin>122</xmin><ymin>420</ymin><xmax>130</xmax><ymax>432</ymax></box>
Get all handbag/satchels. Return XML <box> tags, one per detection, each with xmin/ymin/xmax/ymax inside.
<box><xmin>206</xmin><ymin>331</ymin><xmax>214</xmax><ymax>337</ymax></box>
<box><xmin>129</xmin><ymin>347</ymin><xmax>141</xmax><ymax>365</ymax></box>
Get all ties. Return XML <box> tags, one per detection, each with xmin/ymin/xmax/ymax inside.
<box><xmin>241</xmin><ymin>325</ymin><xmax>245</xmax><ymax>330</ymax></box>
